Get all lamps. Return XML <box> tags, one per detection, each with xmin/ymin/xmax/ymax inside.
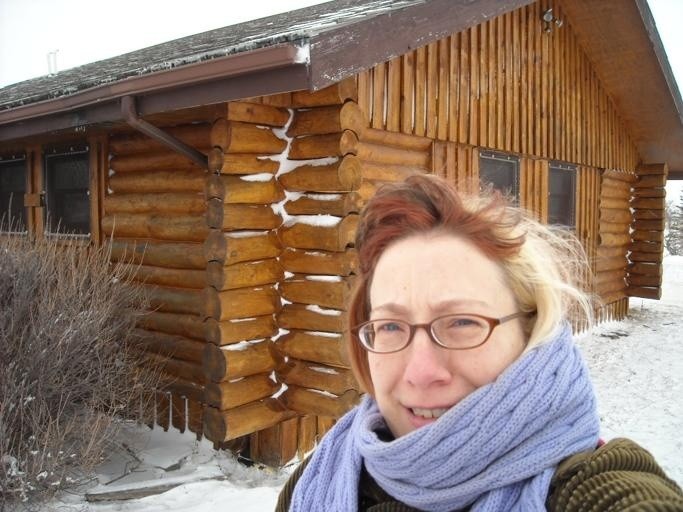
<box><xmin>540</xmin><ymin>7</ymin><xmax>563</xmax><ymax>34</ymax></box>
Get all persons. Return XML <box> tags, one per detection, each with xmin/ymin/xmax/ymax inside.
<box><xmin>273</xmin><ymin>170</ymin><xmax>682</xmax><ymax>511</ymax></box>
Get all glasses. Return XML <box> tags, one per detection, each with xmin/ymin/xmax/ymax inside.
<box><xmin>349</xmin><ymin>309</ymin><xmax>538</xmax><ymax>354</ymax></box>
